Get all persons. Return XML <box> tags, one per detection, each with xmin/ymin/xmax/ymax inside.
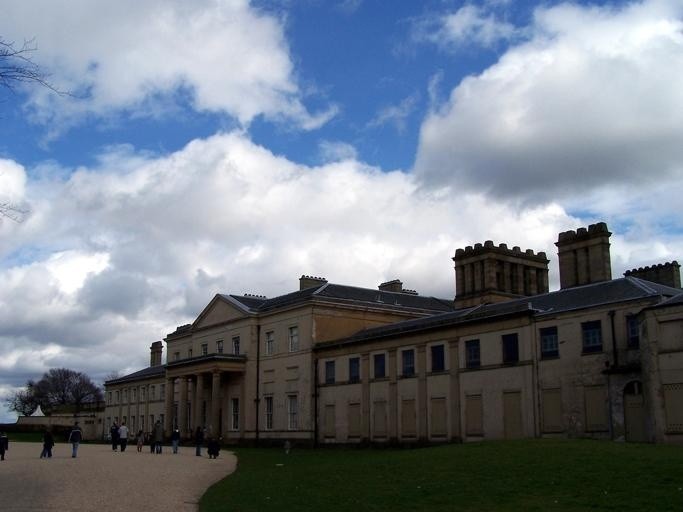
<box><xmin>0</xmin><ymin>431</ymin><xmax>9</xmax><ymax>461</ymax></box>
<box><xmin>66</xmin><ymin>421</ymin><xmax>84</xmax><ymax>458</ymax></box>
<box><xmin>110</xmin><ymin>420</ymin><xmax>130</xmax><ymax>451</ymax></box>
<box><xmin>188</xmin><ymin>424</ymin><xmax>225</xmax><ymax>459</ymax></box>
<box><xmin>40</xmin><ymin>427</ymin><xmax>55</xmax><ymax>458</ymax></box>
<box><xmin>136</xmin><ymin>419</ymin><xmax>164</xmax><ymax>454</ymax></box>
<box><xmin>170</xmin><ymin>425</ymin><xmax>181</xmax><ymax>454</ymax></box>
